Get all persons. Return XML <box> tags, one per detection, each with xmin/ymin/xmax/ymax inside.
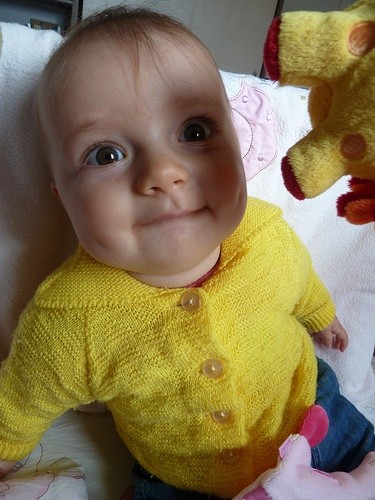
<box><xmin>0</xmin><ymin>5</ymin><xmax>375</xmax><ymax>500</ymax></box>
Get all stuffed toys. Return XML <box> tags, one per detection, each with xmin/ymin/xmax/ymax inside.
<box><xmin>234</xmin><ymin>404</ymin><xmax>375</xmax><ymax>500</ymax></box>
<box><xmin>263</xmin><ymin>0</ymin><xmax>375</xmax><ymax>226</ymax></box>
